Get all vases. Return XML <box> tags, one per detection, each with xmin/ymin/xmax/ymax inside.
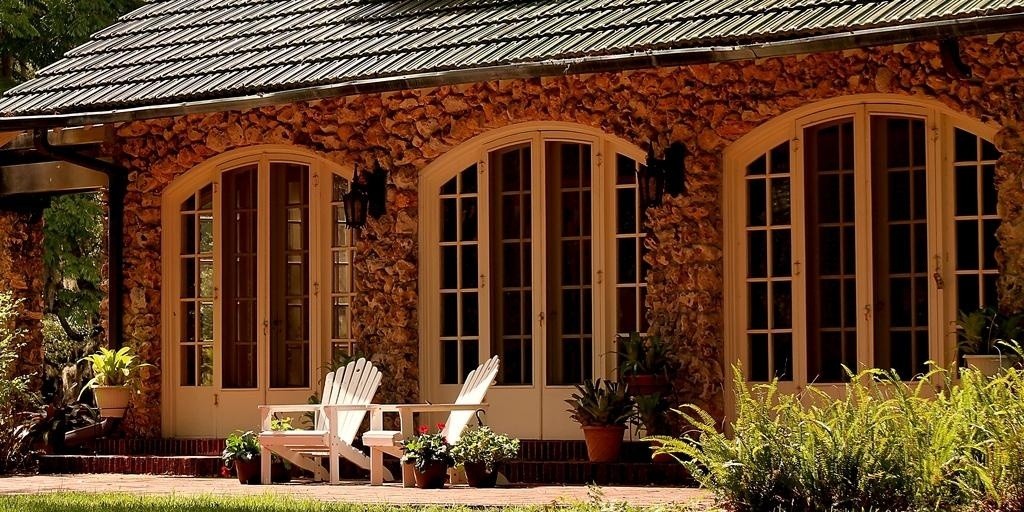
<box><xmin>413</xmin><ymin>461</ymin><xmax>448</xmax><ymax>489</ymax></box>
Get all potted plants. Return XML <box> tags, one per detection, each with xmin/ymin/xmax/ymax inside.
<box><xmin>563</xmin><ymin>317</ymin><xmax>681</xmax><ymax>463</ymax></box>
<box><xmin>73</xmin><ymin>346</ymin><xmax>158</xmax><ymax>418</ymax></box>
<box><xmin>948</xmin><ymin>305</ymin><xmax>1024</xmax><ymax>380</ymax></box>
<box><xmin>450</xmin><ymin>422</ymin><xmax>520</xmax><ymax>486</ymax></box>
<box><xmin>222</xmin><ymin>427</ymin><xmax>259</xmax><ymax>485</ymax></box>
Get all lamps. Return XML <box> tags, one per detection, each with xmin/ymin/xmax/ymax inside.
<box><xmin>342</xmin><ymin>161</ymin><xmax>386</xmax><ymax>231</ymax></box>
<box><xmin>638</xmin><ymin>136</ymin><xmax>689</xmax><ymax>209</ymax></box>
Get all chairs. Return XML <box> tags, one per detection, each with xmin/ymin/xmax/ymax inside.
<box><xmin>255</xmin><ymin>354</ymin><xmax>499</xmax><ymax>487</ymax></box>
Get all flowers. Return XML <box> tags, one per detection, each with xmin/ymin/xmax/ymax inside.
<box><xmin>396</xmin><ymin>422</ymin><xmax>454</xmax><ymax>475</ymax></box>
